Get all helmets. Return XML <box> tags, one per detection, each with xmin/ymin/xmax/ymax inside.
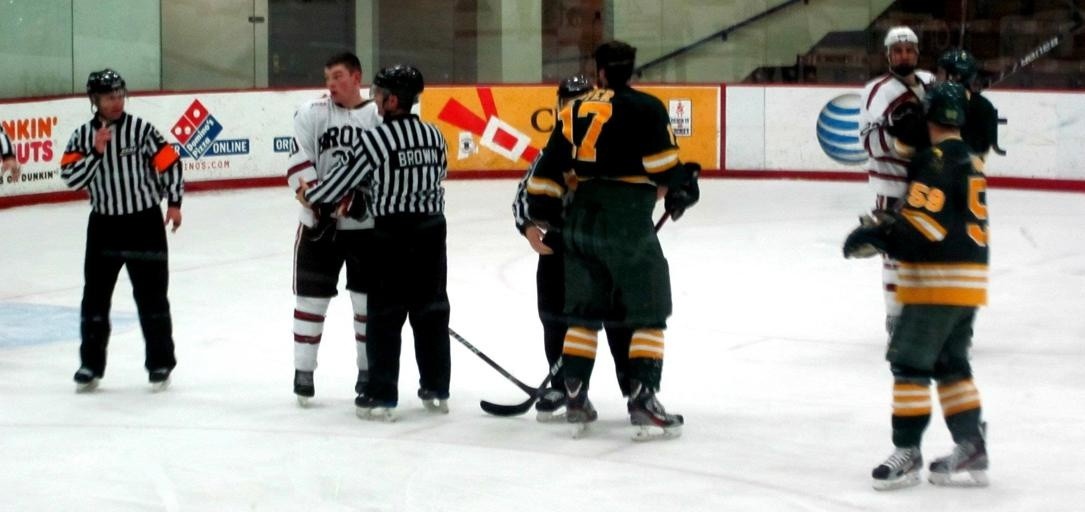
<box><xmin>923</xmin><ymin>80</ymin><xmax>966</xmax><ymax>127</ymax></box>
<box><xmin>936</xmin><ymin>47</ymin><xmax>978</xmax><ymax>86</ymax></box>
<box><xmin>592</xmin><ymin>39</ymin><xmax>635</xmax><ymax>65</ymax></box>
<box><xmin>86</xmin><ymin>68</ymin><xmax>126</xmax><ymax>95</ymax></box>
<box><xmin>558</xmin><ymin>72</ymin><xmax>594</xmax><ymax>96</ymax></box>
<box><xmin>368</xmin><ymin>64</ymin><xmax>424</xmax><ymax>97</ymax></box>
<box><xmin>883</xmin><ymin>25</ymin><xmax>919</xmax><ymax>46</ymax></box>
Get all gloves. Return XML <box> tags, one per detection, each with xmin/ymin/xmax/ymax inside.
<box><xmin>664</xmin><ymin>177</ymin><xmax>700</xmax><ymax>220</ymax></box>
<box><xmin>844</xmin><ymin>221</ymin><xmax>888</xmax><ymax>257</ymax></box>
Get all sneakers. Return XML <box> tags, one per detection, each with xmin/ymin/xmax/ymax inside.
<box><xmin>536</xmin><ymin>389</ymin><xmax>565</xmax><ymax>411</ymax></box>
<box><xmin>354</xmin><ymin>395</ymin><xmax>398</xmax><ymax>407</ymax></box>
<box><xmin>928</xmin><ymin>437</ymin><xmax>987</xmax><ymax>472</ymax></box>
<box><xmin>148</xmin><ymin>365</ymin><xmax>169</xmax><ymax>381</ymax></box>
<box><xmin>627</xmin><ymin>381</ymin><xmax>684</xmax><ymax>427</ymax></box>
<box><xmin>354</xmin><ymin>370</ymin><xmax>368</xmax><ymax>393</ymax></box>
<box><xmin>72</xmin><ymin>365</ymin><xmax>95</xmax><ymax>382</ymax></box>
<box><xmin>562</xmin><ymin>376</ymin><xmax>597</xmax><ymax>422</ymax></box>
<box><xmin>871</xmin><ymin>445</ymin><xmax>924</xmax><ymax>479</ymax></box>
<box><xmin>417</xmin><ymin>387</ymin><xmax>450</xmax><ymax>399</ymax></box>
<box><xmin>292</xmin><ymin>369</ymin><xmax>314</xmax><ymax>395</ymax></box>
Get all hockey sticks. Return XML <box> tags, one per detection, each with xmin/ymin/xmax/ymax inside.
<box><xmin>481</xmin><ymin>209</ymin><xmax>672</xmax><ymax>416</ymax></box>
<box><xmin>448</xmin><ymin>325</ymin><xmax>563</xmax><ymax>400</ymax></box>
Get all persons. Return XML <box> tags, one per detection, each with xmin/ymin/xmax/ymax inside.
<box><xmin>514</xmin><ymin>72</ymin><xmax>634</xmax><ymax>421</ymax></box>
<box><xmin>525</xmin><ymin>40</ymin><xmax>699</xmax><ymax>440</ymax></box>
<box><xmin>857</xmin><ymin>24</ymin><xmax>938</xmax><ymax>334</ymax></box>
<box><xmin>845</xmin><ymin>83</ymin><xmax>993</xmax><ymax>489</ymax></box>
<box><xmin>287</xmin><ymin>54</ymin><xmax>385</xmax><ymax>413</ymax></box>
<box><xmin>931</xmin><ymin>47</ymin><xmax>1006</xmax><ymax>166</ymax></box>
<box><xmin>63</xmin><ymin>71</ymin><xmax>183</xmax><ymax>393</ymax></box>
<box><xmin>296</xmin><ymin>66</ymin><xmax>450</xmax><ymax>418</ymax></box>
